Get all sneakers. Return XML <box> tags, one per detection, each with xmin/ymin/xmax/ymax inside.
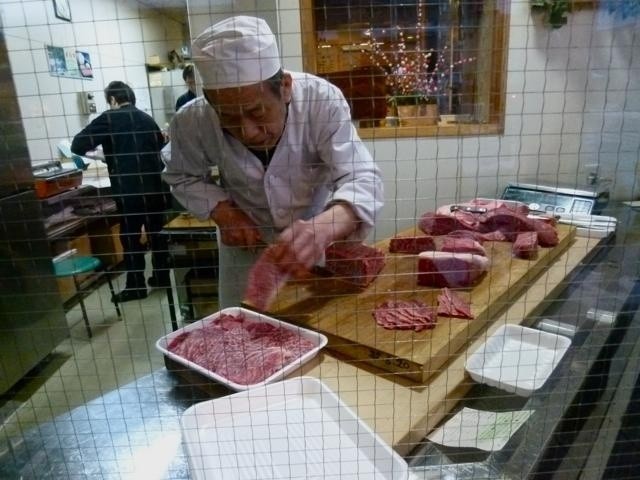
<box><xmin>111</xmin><ymin>290</ymin><xmax>148</xmax><ymax>303</ymax></box>
<box><xmin>148</xmin><ymin>276</ymin><xmax>171</xmax><ymax>286</ymax></box>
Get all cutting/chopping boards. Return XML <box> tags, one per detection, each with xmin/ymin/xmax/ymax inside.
<box><xmin>240</xmin><ymin>223</ymin><xmax>576</xmax><ymax>384</ymax></box>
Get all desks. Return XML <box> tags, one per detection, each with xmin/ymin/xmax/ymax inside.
<box><xmin>157</xmin><ymin>228</ymin><xmax>217</xmax><ymax>331</ymax></box>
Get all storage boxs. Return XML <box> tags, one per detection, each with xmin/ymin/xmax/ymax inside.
<box><xmin>92</xmin><ymin>223</ymin><xmax>148</xmax><ymax>265</ymax></box>
<box><xmin>317</xmin><ymin>44</ymin><xmax>338</xmax><ymax>74</ymax></box>
<box><xmin>191</xmin><ymin>279</ymin><xmax>219</xmax><ymax>319</ymax></box>
<box><xmin>55</xmin><ymin>275</ymin><xmax>78</xmax><ymax>305</ymax></box>
<box><xmin>54</xmin><ymin>234</ymin><xmax>96</xmax><ymax>285</ymax></box>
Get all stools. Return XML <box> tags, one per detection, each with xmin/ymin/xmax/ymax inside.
<box><xmin>53</xmin><ymin>256</ymin><xmax>122</xmax><ymax>338</ymax></box>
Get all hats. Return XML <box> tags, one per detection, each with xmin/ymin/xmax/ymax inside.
<box><xmin>189</xmin><ymin>15</ymin><xmax>281</xmax><ymax>91</ymax></box>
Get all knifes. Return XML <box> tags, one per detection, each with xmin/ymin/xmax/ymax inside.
<box><xmin>451</xmin><ymin>205</ymin><xmax>546</xmax><ymax>215</ymax></box>
<box><xmin>250</xmin><ymin>237</ymin><xmax>334</xmax><ymax>278</ymax></box>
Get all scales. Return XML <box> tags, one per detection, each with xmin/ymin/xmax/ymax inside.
<box><xmin>500</xmin><ymin>178</ymin><xmax>610</xmax><ymax>215</ymax></box>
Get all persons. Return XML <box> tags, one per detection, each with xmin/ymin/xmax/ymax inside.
<box><xmin>175</xmin><ymin>65</ymin><xmax>199</xmax><ymax>112</ymax></box>
<box><xmin>157</xmin><ymin>14</ymin><xmax>387</xmax><ymax>314</ymax></box>
<box><xmin>70</xmin><ymin>82</ymin><xmax>171</xmax><ymax>303</ymax></box>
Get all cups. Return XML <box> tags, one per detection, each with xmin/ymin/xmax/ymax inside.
<box><xmin>385</xmin><ymin>116</ymin><xmax>398</xmax><ymax>128</ymax></box>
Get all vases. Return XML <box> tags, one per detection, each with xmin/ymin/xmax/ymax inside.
<box><xmin>397</xmin><ymin>104</ymin><xmax>440</xmax><ymax>127</ymax></box>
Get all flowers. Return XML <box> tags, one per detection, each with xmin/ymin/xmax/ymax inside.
<box><xmin>357</xmin><ymin>0</ymin><xmax>479</xmax><ymax>106</ymax></box>
